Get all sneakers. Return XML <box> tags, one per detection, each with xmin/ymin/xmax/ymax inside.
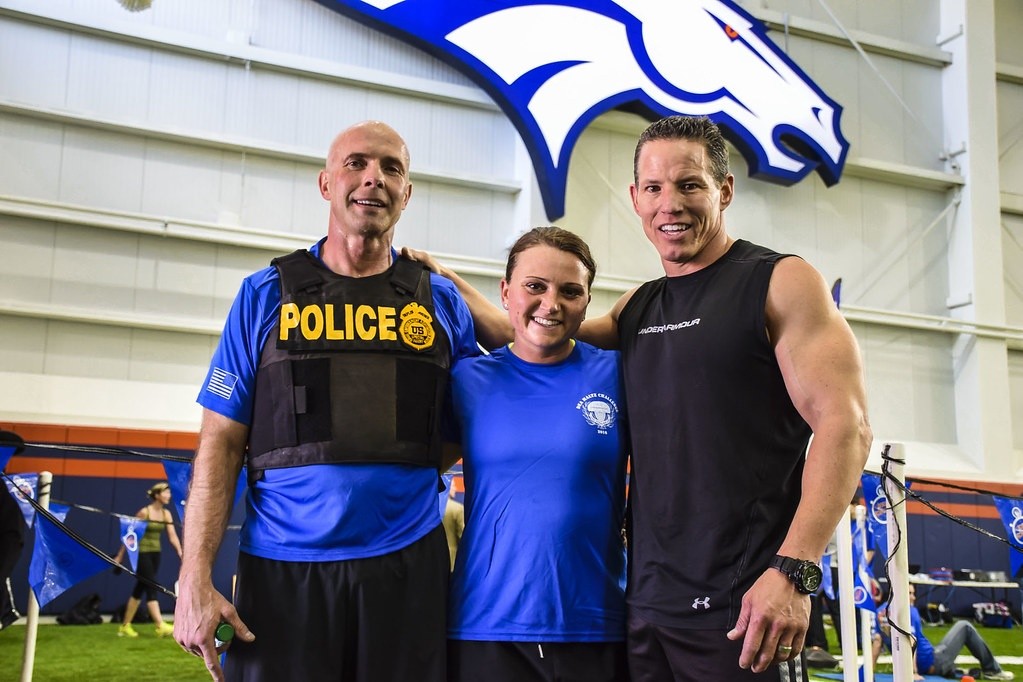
<box><xmin>155</xmin><ymin>620</ymin><xmax>173</xmax><ymax>637</ymax></box>
<box><xmin>118</xmin><ymin>623</ymin><xmax>138</xmax><ymax>637</ymax></box>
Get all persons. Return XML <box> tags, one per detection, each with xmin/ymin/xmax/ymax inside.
<box><xmin>872</xmin><ymin>583</ymin><xmax>1015</xmax><ymax>680</ymax></box>
<box><xmin>114</xmin><ymin>483</ymin><xmax>182</xmax><ymax>639</ymax></box>
<box><xmin>174</xmin><ymin>116</ymin><xmax>879</xmax><ymax>682</ymax></box>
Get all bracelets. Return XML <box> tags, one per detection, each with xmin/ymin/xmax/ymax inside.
<box><xmin>914</xmin><ymin>670</ymin><xmax>918</xmax><ymax>674</ymax></box>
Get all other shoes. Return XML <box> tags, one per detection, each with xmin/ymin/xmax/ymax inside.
<box><xmin>956</xmin><ymin>667</ymin><xmax>968</xmax><ymax>674</ymax></box>
<box><xmin>984</xmin><ymin>670</ymin><xmax>1013</xmax><ymax>680</ymax></box>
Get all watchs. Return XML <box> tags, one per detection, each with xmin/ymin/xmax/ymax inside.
<box><xmin>768</xmin><ymin>553</ymin><xmax>824</xmax><ymax>596</ymax></box>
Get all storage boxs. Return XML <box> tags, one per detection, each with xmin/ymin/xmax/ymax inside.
<box><xmin>983</xmin><ymin>613</ymin><xmax>1013</xmax><ymax>629</ymax></box>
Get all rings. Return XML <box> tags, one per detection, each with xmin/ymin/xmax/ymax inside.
<box><xmin>778</xmin><ymin>645</ymin><xmax>792</xmax><ymax>654</ymax></box>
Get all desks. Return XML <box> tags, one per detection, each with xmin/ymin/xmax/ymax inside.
<box><xmin>880</xmin><ymin>579</ymin><xmax>1018</xmax><ymax>628</ymax></box>
